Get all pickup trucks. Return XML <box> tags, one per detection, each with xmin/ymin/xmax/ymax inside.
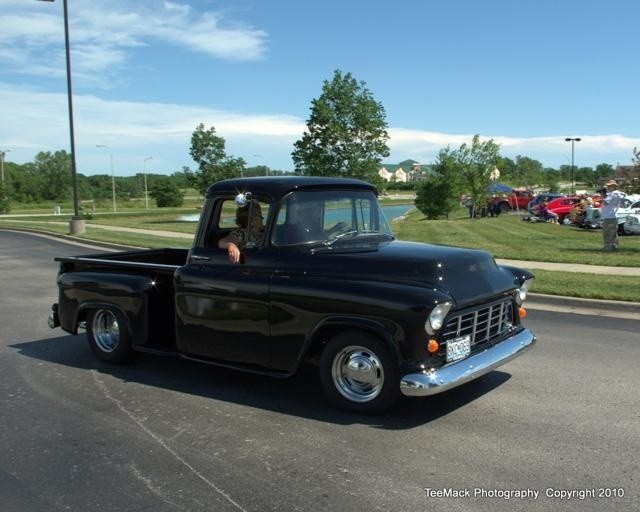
<box><xmin>47</xmin><ymin>175</ymin><xmax>538</xmax><ymax>415</ymax></box>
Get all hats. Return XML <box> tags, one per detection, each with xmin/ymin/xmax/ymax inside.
<box><xmin>605</xmin><ymin>179</ymin><xmax>618</xmax><ymax>187</ymax></box>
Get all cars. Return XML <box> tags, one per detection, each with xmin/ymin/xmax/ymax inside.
<box><xmin>461</xmin><ymin>183</ymin><xmax>640</xmax><ymax>238</ymax></box>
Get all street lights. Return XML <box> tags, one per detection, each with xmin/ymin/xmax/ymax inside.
<box><xmin>0</xmin><ymin>148</ymin><xmax>12</xmax><ymax>184</ymax></box>
<box><xmin>37</xmin><ymin>0</ymin><xmax>87</xmax><ymax>236</ymax></box>
<box><xmin>565</xmin><ymin>137</ymin><xmax>583</xmax><ymax>194</ymax></box>
<box><xmin>144</xmin><ymin>157</ymin><xmax>155</xmax><ymax>209</ymax></box>
<box><xmin>95</xmin><ymin>144</ymin><xmax>118</xmax><ymax>212</ymax></box>
<box><xmin>254</xmin><ymin>154</ymin><xmax>269</xmax><ymax>176</ymax></box>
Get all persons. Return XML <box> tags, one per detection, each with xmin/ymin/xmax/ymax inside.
<box><xmin>540</xmin><ymin>202</ymin><xmax>560</xmax><ymax>225</ymax></box>
<box><xmin>284</xmin><ymin>204</ymin><xmax>322</xmax><ymax>242</ymax></box>
<box><xmin>597</xmin><ymin>179</ymin><xmax>621</xmax><ymax>251</ymax></box>
<box><xmin>486</xmin><ymin>196</ymin><xmax>496</xmax><ymax>217</ymax></box>
<box><xmin>216</xmin><ymin>202</ymin><xmax>267</xmax><ymax>265</ymax></box>
